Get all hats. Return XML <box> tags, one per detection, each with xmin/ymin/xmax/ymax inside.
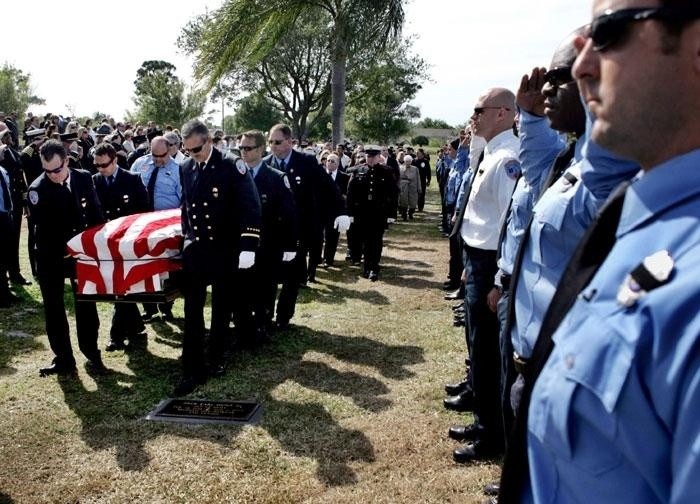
<box><xmin>59</xmin><ymin>132</ymin><xmax>78</xmax><ymax>140</ymax></box>
<box><xmin>362</xmin><ymin>145</ymin><xmax>382</xmax><ymax>155</ymax></box>
<box><xmin>129</xmin><ymin>135</ymin><xmax>146</xmax><ymax>142</ymax></box>
<box><xmin>23</xmin><ymin>128</ymin><xmax>46</xmax><ymax>139</ymax></box>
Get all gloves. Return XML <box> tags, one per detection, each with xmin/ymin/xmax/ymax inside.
<box><xmin>388</xmin><ymin>218</ymin><xmax>396</xmax><ymax>224</ymax></box>
<box><xmin>333</xmin><ymin>215</ymin><xmax>351</xmax><ymax>234</ymax></box>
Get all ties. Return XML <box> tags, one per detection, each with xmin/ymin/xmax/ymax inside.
<box><xmin>148</xmin><ymin>167</ymin><xmax>160</xmax><ymax>212</ymax></box>
<box><xmin>448</xmin><ymin>150</ymin><xmax>484</xmax><ymax>240</ymax></box>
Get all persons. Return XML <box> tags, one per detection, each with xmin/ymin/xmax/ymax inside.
<box><xmin>1</xmin><ymin>113</ymin><xmax>430</xmax><ymax>399</ymax></box>
<box><xmin>435</xmin><ymin>1</ymin><xmax>699</xmax><ymax>503</ymax></box>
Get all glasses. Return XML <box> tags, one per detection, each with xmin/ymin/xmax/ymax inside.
<box><xmin>42</xmin><ymin>139</ymin><xmax>284</xmax><ymax>174</ymax></box>
<box><xmin>475</xmin><ymin>107</ymin><xmax>510</xmax><ymax>115</ymax></box>
<box><xmin>544</xmin><ymin>66</ymin><xmax>573</xmax><ymax>87</ymax></box>
<box><xmin>586</xmin><ymin>5</ymin><xmax>662</xmax><ymax>52</ymax></box>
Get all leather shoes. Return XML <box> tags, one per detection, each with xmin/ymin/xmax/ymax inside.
<box><xmin>438</xmin><ymin>210</ymin><xmax>501</xmax><ymax>495</ymax></box>
